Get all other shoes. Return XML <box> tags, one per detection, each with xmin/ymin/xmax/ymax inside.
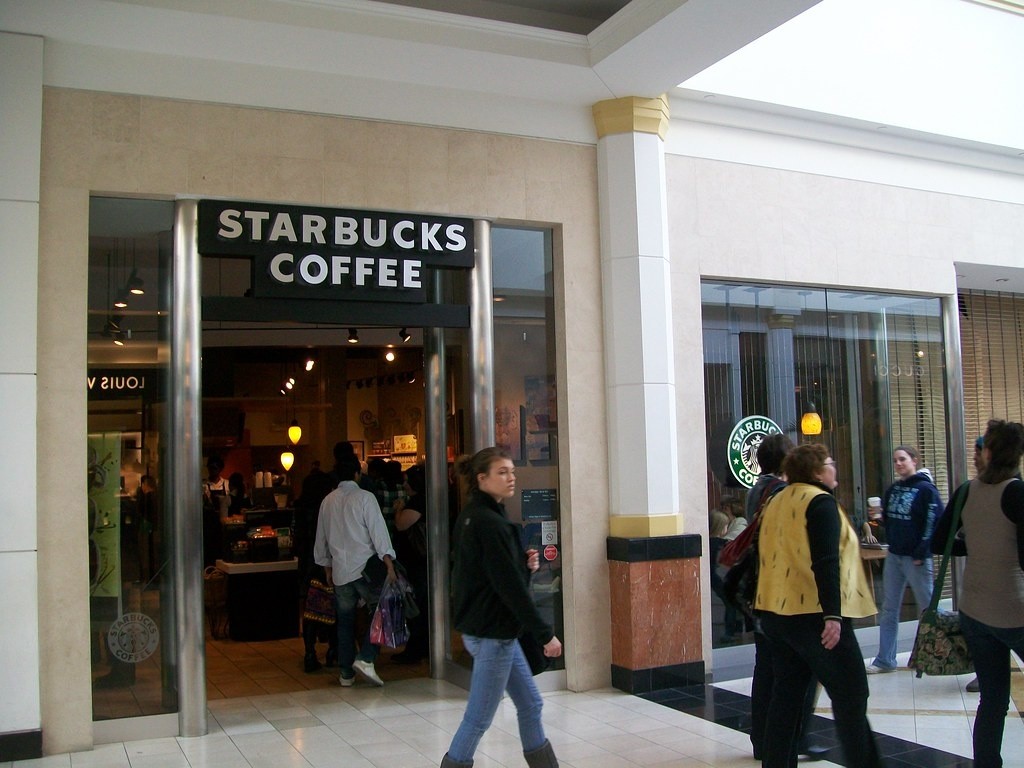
<box><xmin>965</xmin><ymin>676</ymin><xmax>981</xmax><ymax>693</ymax></box>
<box><xmin>866</xmin><ymin>665</ymin><xmax>897</xmax><ymax>673</ymax></box>
<box><xmin>719</xmin><ymin>633</ymin><xmax>742</xmax><ymax>644</ymax></box>
<box><xmin>795</xmin><ymin>741</ymin><xmax>832</xmax><ymax>759</ymax></box>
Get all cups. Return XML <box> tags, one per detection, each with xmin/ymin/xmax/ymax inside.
<box><xmin>868</xmin><ymin>496</ymin><xmax>882</xmax><ymax>520</ymax></box>
<box><xmin>255</xmin><ymin>471</ymin><xmax>287</xmax><ymax>508</ymax></box>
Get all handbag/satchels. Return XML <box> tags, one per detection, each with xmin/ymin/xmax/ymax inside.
<box><xmin>718</xmin><ymin>479</ymin><xmax>781</xmax><ymax>567</ymax></box>
<box><xmin>370</xmin><ymin>572</ymin><xmax>419</xmax><ymax>648</ymax></box>
<box><xmin>907</xmin><ymin>607</ymin><xmax>977</xmax><ymax>676</ymax></box>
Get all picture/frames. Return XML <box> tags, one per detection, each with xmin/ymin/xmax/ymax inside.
<box><xmin>350</xmin><ymin>440</ymin><xmax>365</xmax><ymax>461</ymax></box>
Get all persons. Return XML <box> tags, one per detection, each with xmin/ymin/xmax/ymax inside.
<box><xmin>311</xmin><ymin>455</ymin><xmax>398</xmax><ymax>686</ymax></box>
<box><xmin>708</xmin><ymin>496</ymin><xmax>753</xmax><ymax>631</ymax></box>
<box><xmin>965</xmin><ymin>437</ymin><xmax>1024</xmax><ymax>692</ymax></box>
<box><xmin>130</xmin><ymin>439</ymin><xmax>428</xmax><ymax>686</ymax></box>
<box><xmin>743</xmin><ymin>434</ymin><xmax>833</xmax><ymax>761</ymax></box>
<box><xmin>754</xmin><ymin>443</ymin><xmax>882</xmax><ymax>768</ymax></box>
<box><xmin>866</xmin><ymin>446</ymin><xmax>945</xmax><ymax>674</ymax></box>
<box><xmin>439</xmin><ymin>447</ymin><xmax>561</xmax><ymax>768</ymax></box>
<box><xmin>930</xmin><ymin>422</ymin><xmax>1024</xmax><ymax>768</ymax></box>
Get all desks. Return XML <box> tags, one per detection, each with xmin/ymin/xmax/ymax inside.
<box><xmin>216</xmin><ymin>556</ymin><xmax>303</xmax><ymax>641</ymax></box>
<box><xmin>860</xmin><ymin>548</ymin><xmax>887</xmax><ymax>625</ymax></box>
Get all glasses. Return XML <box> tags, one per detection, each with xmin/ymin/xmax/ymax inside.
<box><xmin>819</xmin><ymin>460</ymin><xmax>837</xmax><ymax>467</ymax></box>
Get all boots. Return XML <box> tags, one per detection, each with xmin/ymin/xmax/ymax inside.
<box><xmin>440</xmin><ymin>752</ymin><xmax>474</xmax><ymax>768</ymax></box>
<box><xmin>523</xmin><ymin>738</ymin><xmax>559</xmax><ymax>768</ymax></box>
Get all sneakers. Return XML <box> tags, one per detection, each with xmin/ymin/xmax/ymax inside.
<box><xmin>352</xmin><ymin>659</ymin><xmax>384</xmax><ymax>686</ymax></box>
<box><xmin>339</xmin><ymin>673</ymin><xmax>355</xmax><ymax>686</ymax></box>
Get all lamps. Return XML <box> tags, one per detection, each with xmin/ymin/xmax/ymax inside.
<box><xmin>304</xmin><ymin>351</ymin><xmax>315</xmax><ymax>373</ymax></box>
<box><xmin>346</xmin><ymin>328</ymin><xmax>360</xmax><ymax>343</ymax></box>
<box><xmin>280</xmin><ymin>397</ymin><xmax>294</xmax><ymax>471</ymax></box>
<box><xmin>801</xmin><ymin>363</ymin><xmax>827</xmax><ymax>436</ymax></box>
<box><xmin>344</xmin><ymin>372</ymin><xmax>417</xmax><ymax>389</ymax></box>
<box><xmin>103</xmin><ymin>237</ymin><xmax>144</xmax><ymax>346</ymax></box>
<box><xmin>287</xmin><ymin>394</ymin><xmax>302</xmax><ymax>444</ymax></box>
<box><xmin>276</xmin><ymin>357</ymin><xmax>295</xmax><ymax>394</ymax></box>
<box><xmin>399</xmin><ymin>328</ymin><xmax>411</xmax><ymax>344</ymax></box>
